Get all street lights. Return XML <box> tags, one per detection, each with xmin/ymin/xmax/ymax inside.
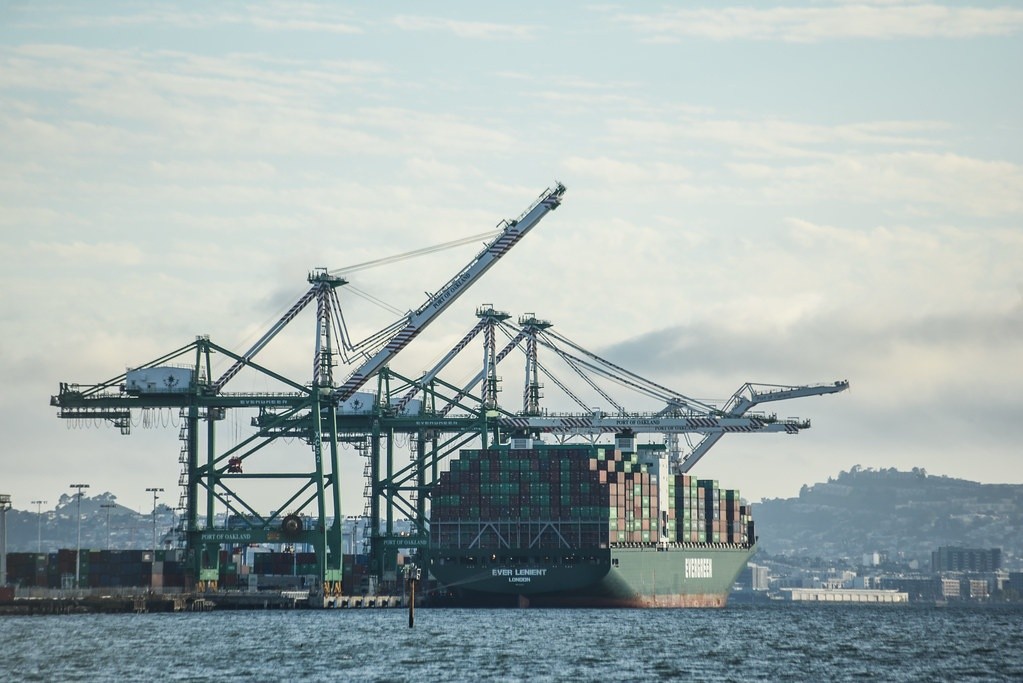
<box><xmin>69</xmin><ymin>484</ymin><xmax>90</xmax><ymax>588</ymax></box>
<box><xmin>100</xmin><ymin>505</ymin><xmax>115</xmax><ymax>551</ymax></box>
<box><xmin>166</xmin><ymin>507</ymin><xmax>182</xmax><ymax>549</ymax></box>
<box><xmin>31</xmin><ymin>501</ymin><xmax>47</xmax><ymax>553</ymax></box>
<box><xmin>145</xmin><ymin>488</ymin><xmax>164</xmax><ymax>562</ymax></box>
<box><xmin>218</xmin><ymin>492</ymin><xmax>234</xmax><ymax>531</ymax></box>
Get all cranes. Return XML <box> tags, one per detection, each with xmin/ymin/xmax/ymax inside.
<box><xmin>53</xmin><ymin>182</ymin><xmax>850</xmax><ymax>588</ymax></box>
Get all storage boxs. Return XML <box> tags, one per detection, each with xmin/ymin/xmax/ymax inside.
<box><xmin>429</xmin><ymin>443</ymin><xmax>756</xmax><ymax>547</ymax></box>
<box><xmin>7</xmin><ymin>547</ymin><xmax>368</xmax><ymax>597</ymax></box>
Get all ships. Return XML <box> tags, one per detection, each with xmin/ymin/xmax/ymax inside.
<box><xmin>417</xmin><ymin>432</ymin><xmax>761</xmax><ymax>608</ymax></box>
